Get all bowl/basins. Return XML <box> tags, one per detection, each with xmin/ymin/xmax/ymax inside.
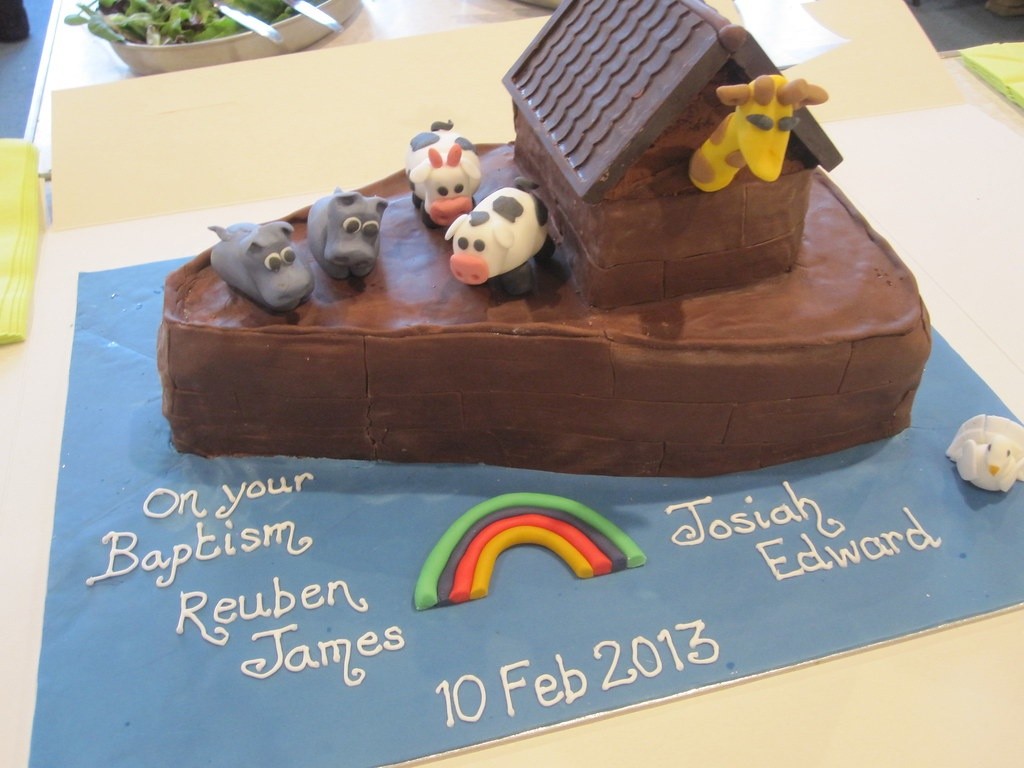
<box><xmin>107</xmin><ymin>1</ymin><xmax>359</xmax><ymax>73</ymax></box>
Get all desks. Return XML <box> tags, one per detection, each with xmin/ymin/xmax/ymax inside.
<box><xmin>1</xmin><ymin>0</ymin><xmax>1022</xmax><ymax>768</ymax></box>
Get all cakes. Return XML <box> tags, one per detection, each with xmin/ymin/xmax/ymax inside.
<box><xmin>157</xmin><ymin>0</ymin><xmax>930</xmax><ymax>476</ymax></box>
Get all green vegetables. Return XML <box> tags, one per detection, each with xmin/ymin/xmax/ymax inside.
<box><xmin>64</xmin><ymin>0</ymin><xmax>310</xmax><ymax>46</ymax></box>
